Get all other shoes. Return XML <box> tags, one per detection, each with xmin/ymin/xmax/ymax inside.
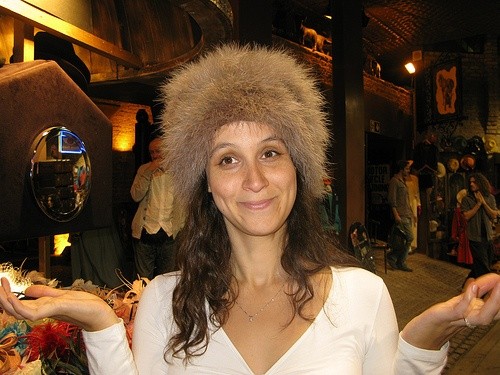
<box><xmin>387</xmin><ymin>252</ymin><xmax>398</xmax><ymax>269</ymax></box>
<box><xmin>398</xmin><ymin>264</ymin><xmax>413</xmax><ymax>272</ymax></box>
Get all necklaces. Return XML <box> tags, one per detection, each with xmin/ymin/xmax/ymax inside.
<box><xmin>234</xmin><ymin>275</ymin><xmax>290</xmax><ymax>322</ymax></box>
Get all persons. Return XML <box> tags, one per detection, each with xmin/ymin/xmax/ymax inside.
<box><xmin>388</xmin><ymin>161</ymin><xmax>417</xmax><ymax>272</ymax></box>
<box><xmin>459</xmin><ymin>172</ymin><xmax>496</xmax><ymax>289</ymax></box>
<box><xmin>0</xmin><ymin>43</ymin><xmax>500</xmax><ymax>375</ymax></box>
<box><xmin>131</xmin><ymin>135</ymin><xmax>179</xmax><ymax>284</ymax></box>
<box><xmin>404</xmin><ymin>173</ymin><xmax>421</xmax><ymax>255</ymax></box>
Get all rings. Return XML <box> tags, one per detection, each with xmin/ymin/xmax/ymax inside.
<box><xmin>463</xmin><ymin>316</ymin><xmax>477</xmax><ymax>328</ymax></box>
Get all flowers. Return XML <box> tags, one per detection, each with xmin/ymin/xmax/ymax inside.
<box><xmin>0</xmin><ymin>259</ymin><xmax>153</xmax><ymax>375</ymax></box>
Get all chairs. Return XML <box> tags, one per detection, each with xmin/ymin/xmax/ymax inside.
<box><xmin>351</xmin><ymin>223</ymin><xmax>389</xmax><ymax>273</ymax></box>
